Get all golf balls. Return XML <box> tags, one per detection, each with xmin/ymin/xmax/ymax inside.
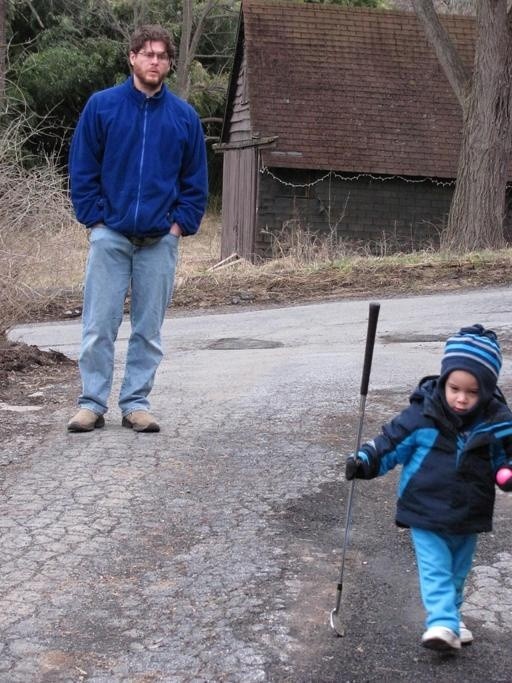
<box><xmin>496</xmin><ymin>468</ymin><xmax>511</xmax><ymax>486</ymax></box>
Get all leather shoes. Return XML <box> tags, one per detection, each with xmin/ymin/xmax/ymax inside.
<box><xmin>68</xmin><ymin>408</ymin><xmax>105</xmax><ymax>431</ymax></box>
<box><xmin>122</xmin><ymin>409</ymin><xmax>160</xmax><ymax>432</ymax></box>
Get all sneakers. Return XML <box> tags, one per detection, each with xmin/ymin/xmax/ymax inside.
<box><xmin>421</xmin><ymin>620</ymin><xmax>473</xmax><ymax>651</ymax></box>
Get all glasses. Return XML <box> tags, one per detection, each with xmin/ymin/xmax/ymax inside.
<box><xmin>138</xmin><ymin>51</ymin><xmax>170</xmax><ymax>61</ymax></box>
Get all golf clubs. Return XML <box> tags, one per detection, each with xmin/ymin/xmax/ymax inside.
<box><xmin>330</xmin><ymin>302</ymin><xmax>380</xmax><ymax>636</ymax></box>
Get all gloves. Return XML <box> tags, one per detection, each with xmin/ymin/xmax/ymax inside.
<box><xmin>345</xmin><ymin>457</ymin><xmax>366</xmax><ymax>481</ymax></box>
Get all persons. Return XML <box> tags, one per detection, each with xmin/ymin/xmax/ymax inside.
<box><xmin>68</xmin><ymin>25</ymin><xmax>209</xmax><ymax>433</ymax></box>
<box><xmin>344</xmin><ymin>323</ymin><xmax>511</xmax><ymax>652</ymax></box>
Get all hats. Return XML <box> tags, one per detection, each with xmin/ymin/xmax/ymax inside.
<box><xmin>437</xmin><ymin>324</ymin><xmax>502</xmax><ymax>403</ymax></box>
